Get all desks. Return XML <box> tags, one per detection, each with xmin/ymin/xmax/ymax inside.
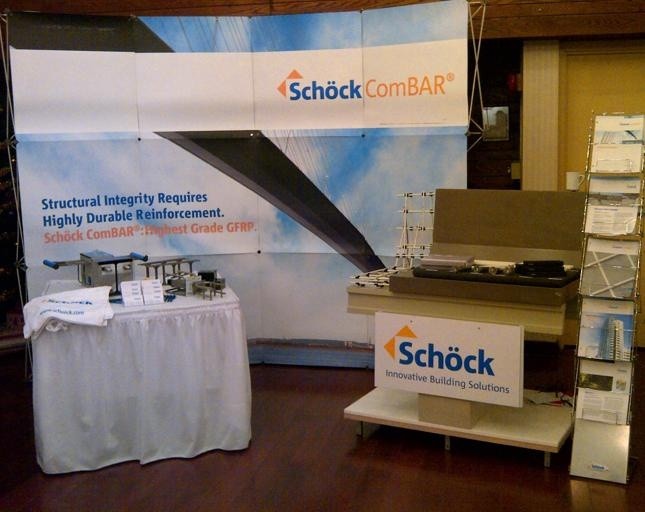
<box><xmin>32</xmin><ymin>271</ymin><xmax>253</xmax><ymax>476</ymax></box>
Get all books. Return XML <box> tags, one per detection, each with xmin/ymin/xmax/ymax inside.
<box><xmin>588</xmin><ymin>113</ymin><xmax>645</xmax><ymax>174</ymax></box>
<box><xmin>583</xmin><ymin>180</ymin><xmax>643</xmax><ymax>236</ymax></box>
<box><xmin>581</xmin><ymin>237</ymin><xmax>643</xmax><ymax>303</ymax></box>
<box><xmin>575</xmin><ymin>360</ymin><xmax>634</xmax><ymax>428</ymax></box>
<box><xmin>577</xmin><ymin>297</ymin><xmax>637</xmax><ymax>365</ymax></box>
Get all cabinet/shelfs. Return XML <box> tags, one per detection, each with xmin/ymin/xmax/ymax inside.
<box><xmin>343</xmin><ymin>279</ymin><xmax>580</xmax><ymax>467</ymax></box>
